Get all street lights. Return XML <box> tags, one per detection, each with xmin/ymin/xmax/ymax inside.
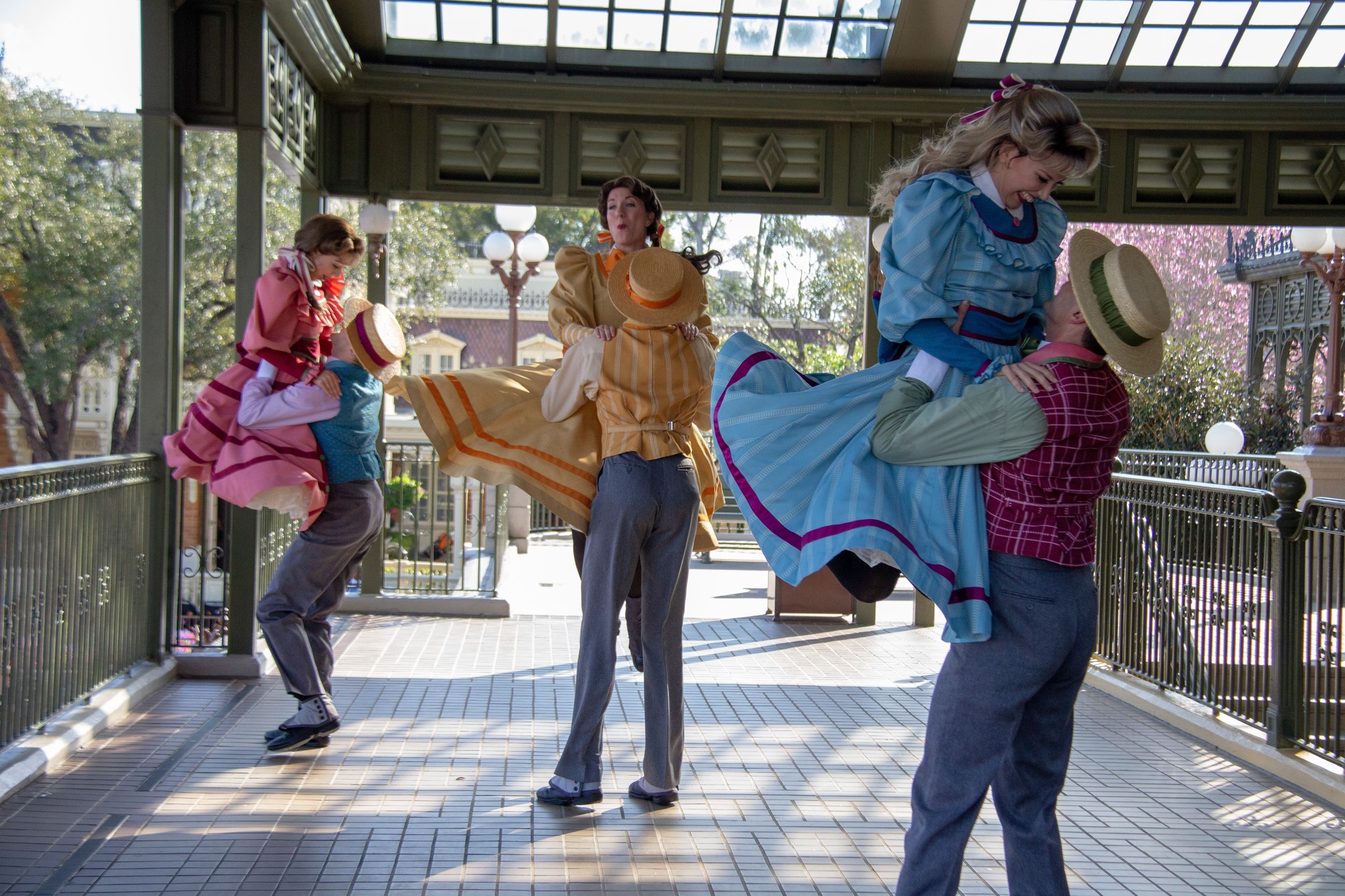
<box><xmin>482</xmin><ymin>204</ymin><xmax>550</xmax><ymax>368</ymax></box>
<box><xmin>1291</xmin><ymin>228</ymin><xmax>1345</xmax><ymax>449</ymax></box>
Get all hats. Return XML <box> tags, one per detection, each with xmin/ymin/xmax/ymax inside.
<box><xmin>608</xmin><ymin>247</ymin><xmax>703</xmax><ymax>327</ymax></box>
<box><xmin>1068</xmin><ymin>228</ymin><xmax>1172</xmax><ymax>378</ymax></box>
<box><xmin>343</xmin><ymin>295</ymin><xmax>406</xmax><ymax>384</ymax></box>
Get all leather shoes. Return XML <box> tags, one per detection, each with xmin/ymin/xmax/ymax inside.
<box><xmin>267</xmin><ymin>720</ymin><xmax>340</xmax><ymax>752</ymax></box>
<box><xmin>627</xmin><ymin>781</ymin><xmax>680</xmax><ymax>803</ymax></box>
<box><xmin>537</xmin><ymin>784</ymin><xmax>602</xmax><ymax>806</ymax></box>
<box><xmin>264</xmin><ymin>730</ymin><xmax>330</xmax><ymax>746</ymax></box>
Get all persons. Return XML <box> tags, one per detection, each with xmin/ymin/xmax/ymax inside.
<box><xmin>537</xmin><ymin>244</ymin><xmax>716</xmax><ymax>809</ymax></box>
<box><xmin>177</xmin><ymin>606</ymin><xmax>229</xmax><ymax>653</ymax></box>
<box><xmin>873</xmin><ymin>226</ymin><xmax>1168</xmax><ymax>896</ymax></box>
<box><xmin>237</xmin><ymin>296</ymin><xmax>409</xmax><ymax>753</ymax></box>
<box><xmin>389</xmin><ymin>176</ymin><xmax>725</xmax><ymax>672</ymax></box>
<box><xmin>162</xmin><ymin>210</ymin><xmax>366</xmax><ymax>529</ymax></box>
<box><xmin>710</xmin><ymin>71</ymin><xmax>1102</xmax><ymax>644</ymax></box>
